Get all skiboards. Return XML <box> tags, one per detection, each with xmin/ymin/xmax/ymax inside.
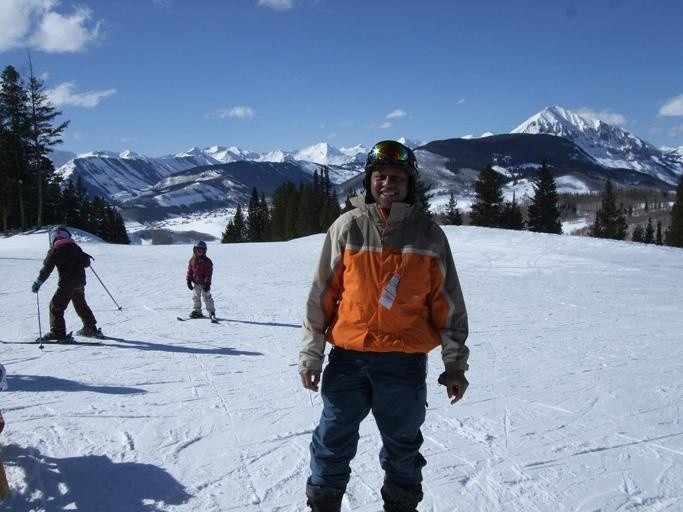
<box><xmin>177</xmin><ymin>317</ymin><xmax>219</xmax><ymax>323</ymax></box>
<box><xmin>0</xmin><ymin>333</ymin><xmax>125</xmax><ymax>346</ymax></box>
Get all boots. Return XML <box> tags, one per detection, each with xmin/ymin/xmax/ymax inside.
<box><xmin>381</xmin><ymin>479</ymin><xmax>423</xmax><ymax>510</ymax></box>
<box><xmin>306</xmin><ymin>476</ymin><xmax>347</xmax><ymax>512</ymax></box>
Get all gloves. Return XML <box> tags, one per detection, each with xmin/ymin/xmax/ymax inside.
<box><xmin>31</xmin><ymin>279</ymin><xmax>43</xmax><ymax>293</ymax></box>
<box><xmin>202</xmin><ymin>285</ymin><xmax>210</xmax><ymax>292</ymax></box>
<box><xmin>187</xmin><ymin>280</ymin><xmax>193</xmax><ymax>290</ymax></box>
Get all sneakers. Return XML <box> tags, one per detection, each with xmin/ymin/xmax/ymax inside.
<box><xmin>189</xmin><ymin>311</ymin><xmax>203</xmax><ymax>318</ymax></box>
<box><xmin>77</xmin><ymin>325</ymin><xmax>97</xmax><ymax>334</ymax></box>
<box><xmin>44</xmin><ymin>332</ymin><xmax>67</xmax><ymax>339</ymax></box>
<box><xmin>208</xmin><ymin>312</ymin><xmax>217</xmax><ymax>323</ymax></box>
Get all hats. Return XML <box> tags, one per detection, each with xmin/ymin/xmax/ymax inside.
<box><xmin>193</xmin><ymin>240</ymin><xmax>207</xmax><ymax>249</ymax></box>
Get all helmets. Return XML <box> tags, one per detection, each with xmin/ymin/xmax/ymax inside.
<box><xmin>51</xmin><ymin>227</ymin><xmax>71</xmax><ymax>241</ymax></box>
<box><xmin>365</xmin><ymin>140</ymin><xmax>418</xmax><ymax>181</ymax></box>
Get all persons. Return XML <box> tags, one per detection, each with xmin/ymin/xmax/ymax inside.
<box><xmin>298</xmin><ymin>140</ymin><xmax>470</xmax><ymax>512</ymax></box>
<box><xmin>187</xmin><ymin>240</ymin><xmax>216</xmax><ymax>319</ymax></box>
<box><xmin>31</xmin><ymin>225</ymin><xmax>98</xmax><ymax>339</ymax></box>
